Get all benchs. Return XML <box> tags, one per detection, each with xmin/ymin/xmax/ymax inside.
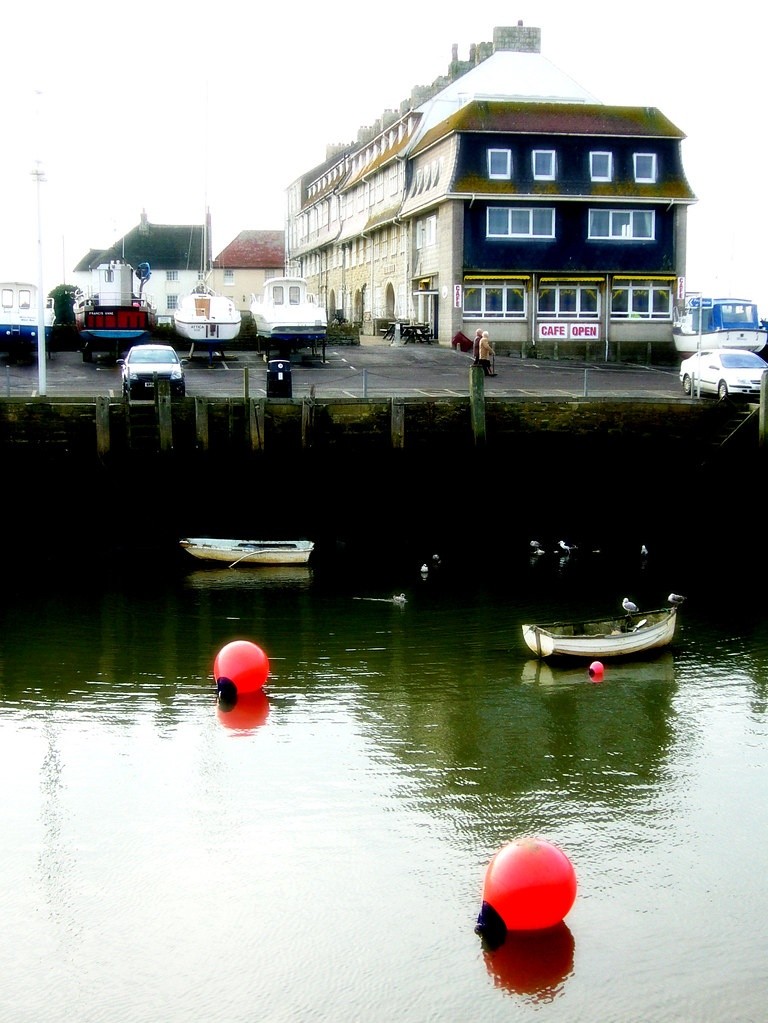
<box><xmin>380</xmin><ymin>329</ymin><xmax>394</xmax><ymax>332</ymax></box>
<box><xmin>401</xmin><ymin>333</ymin><xmax>434</xmax><ymax>337</ymax></box>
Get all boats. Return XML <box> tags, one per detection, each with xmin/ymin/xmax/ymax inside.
<box><xmin>0</xmin><ymin>282</ymin><xmax>56</xmax><ymax>352</ymax></box>
<box><xmin>521</xmin><ymin>650</ymin><xmax>681</xmax><ymax>688</ymax></box>
<box><xmin>183</xmin><ymin>560</ymin><xmax>314</xmax><ymax>593</ymax></box>
<box><xmin>171</xmin><ymin>207</ymin><xmax>241</xmax><ymax>342</ymax></box>
<box><xmin>671</xmin><ymin>296</ymin><xmax>768</xmax><ymax>359</ymax></box>
<box><xmin>250</xmin><ymin>276</ymin><xmax>329</xmax><ymax>340</ymax></box>
<box><xmin>70</xmin><ymin>224</ymin><xmax>156</xmax><ymax>363</ymax></box>
<box><xmin>517</xmin><ymin>606</ymin><xmax>678</xmax><ymax>662</ymax></box>
<box><xmin>177</xmin><ymin>534</ymin><xmax>316</xmax><ymax>564</ymax></box>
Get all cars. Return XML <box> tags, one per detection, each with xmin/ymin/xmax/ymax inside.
<box><xmin>116</xmin><ymin>345</ymin><xmax>189</xmax><ymax>399</ymax></box>
<box><xmin>680</xmin><ymin>349</ymin><xmax>768</xmax><ymax>401</ymax></box>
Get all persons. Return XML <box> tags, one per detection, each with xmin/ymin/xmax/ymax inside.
<box><xmin>471</xmin><ymin>328</ymin><xmax>495</xmax><ymax>376</ymax></box>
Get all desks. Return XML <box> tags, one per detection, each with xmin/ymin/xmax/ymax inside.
<box><xmin>383</xmin><ymin>322</ymin><xmax>410</xmax><ymax>340</ymax></box>
<box><xmin>401</xmin><ymin>325</ymin><xmax>432</xmax><ymax>345</ymax></box>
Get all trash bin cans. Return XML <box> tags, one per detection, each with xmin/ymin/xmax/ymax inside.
<box><xmin>266</xmin><ymin>360</ymin><xmax>292</xmax><ymax>397</ymax></box>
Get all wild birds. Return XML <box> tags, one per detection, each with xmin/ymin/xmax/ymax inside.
<box><xmin>530</xmin><ymin>541</ymin><xmax>542</xmax><ymax>548</ymax></box>
<box><xmin>621</xmin><ymin>597</ymin><xmax>639</xmax><ymax>617</ymax></box>
<box><xmin>668</xmin><ymin>593</ymin><xmax>687</xmax><ymax>609</ymax></box>
<box><xmin>431</xmin><ymin>554</ymin><xmax>443</xmax><ymax>564</ymax></box>
<box><xmin>420</xmin><ymin>564</ymin><xmax>428</xmax><ymax>572</ymax></box>
<box><xmin>558</xmin><ymin>541</ymin><xmax>578</xmax><ymax>551</ymax></box>
<box><xmin>641</xmin><ymin>545</ymin><xmax>648</xmax><ymax>555</ymax></box>
<box><xmin>393</xmin><ymin>593</ymin><xmax>407</xmax><ymax>601</ymax></box>
